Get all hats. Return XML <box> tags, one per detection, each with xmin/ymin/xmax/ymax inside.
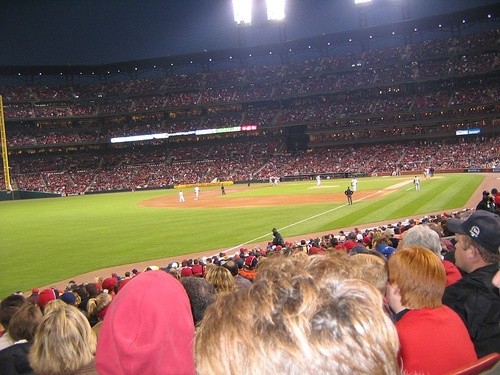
<box><xmin>63</xmin><ymin>292</ymin><xmax>76</xmax><ymax>304</ymax></box>
<box><xmin>446</xmin><ymin>210</ymin><xmax>500</xmax><ymax>248</ymax></box>
<box><xmin>377</xmin><ymin>244</ymin><xmax>395</xmax><ymax>259</ymax></box>
<box><xmin>38</xmin><ymin>289</ymin><xmax>56</xmax><ymax>306</ymax></box>
<box><xmin>101</xmin><ymin>278</ymin><xmax>116</xmax><ymax>290</ymax></box>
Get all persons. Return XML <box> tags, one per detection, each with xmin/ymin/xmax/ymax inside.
<box><xmin>344</xmin><ymin>187</ymin><xmax>354</xmax><ymax>205</ymax></box>
<box><xmin>0</xmin><ymin>27</ymin><xmax>500</xmax><ymax>195</ymax></box>
<box><xmin>0</xmin><ymin>188</ymin><xmax>500</xmax><ymax>375</ymax></box>
